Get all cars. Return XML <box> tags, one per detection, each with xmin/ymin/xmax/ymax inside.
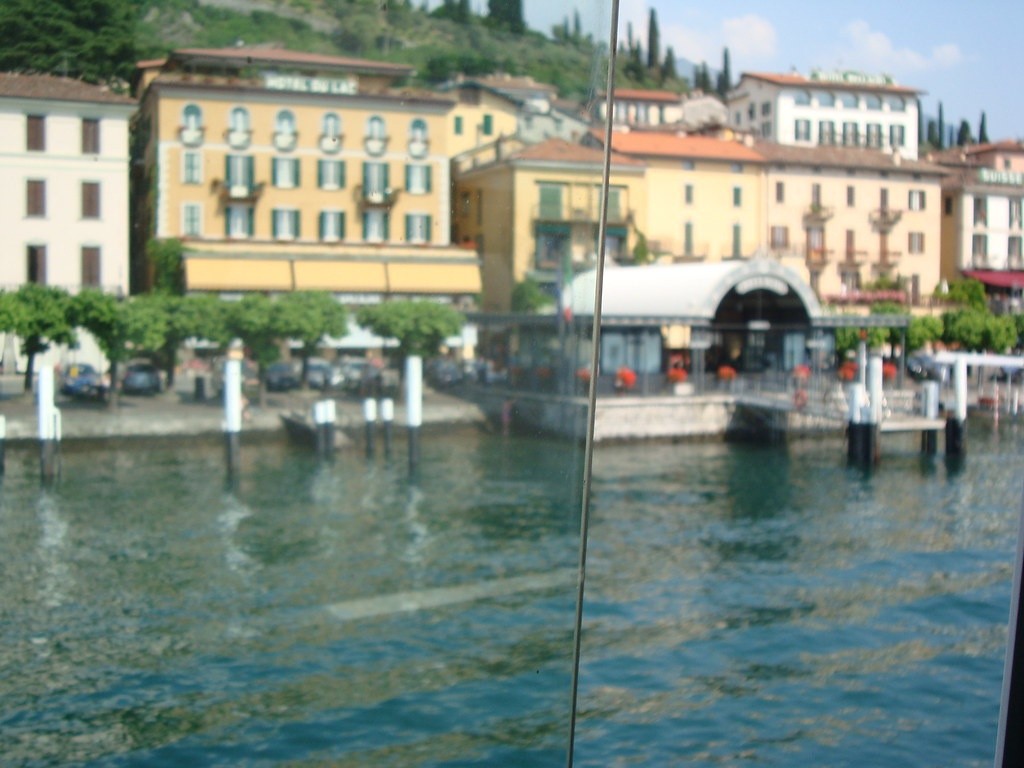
<box><xmin>120</xmin><ymin>363</ymin><xmax>161</xmax><ymax>397</ymax></box>
<box><xmin>213</xmin><ymin>342</ymin><xmax>507</xmax><ymax>397</ymax></box>
<box><xmin>59</xmin><ymin>362</ymin><xmax>102</xmax><ymax>397</ymax></box>
